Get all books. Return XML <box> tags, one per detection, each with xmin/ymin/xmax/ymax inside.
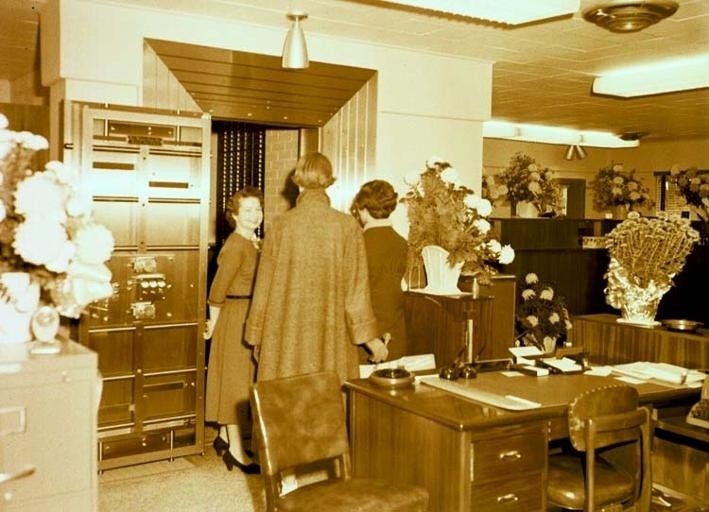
<box><xmin>610</xmin><ymin>357</ymin><xmax>709</xmax><ymax>391</ymax></box>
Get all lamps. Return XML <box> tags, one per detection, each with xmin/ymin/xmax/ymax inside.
<box><xmin>283</xmin><ymin>12</ymin><xmax>308</xmax><ymax>70</ymax></box>
<box><xmin>483</xmin><ymin>0</ymin><xmax>709</xmax><ymax>160</ymax></box>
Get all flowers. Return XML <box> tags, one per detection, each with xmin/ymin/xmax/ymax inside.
<box><xmin>588</xmin><ymin>162</ymin><xmax>709</xmax><ymax>222</ymax></box>
<box><xmin>0</xmin><ymin>114</ymin><xmax>117</xmax><ymax>342</ymax></box>
<box><xmin>398</xmin><ymin>160</ymin><xmax>515</xmax><ymax>286</ymax></box>
<box><xmin>518</xmin><ymin>273</ymin><xmax>574</xmax><ymax>339</ymax></box>
<box><xmin>482</xmin><ymin>151</ymin><xmax>565</xmax><ymax>212</ymax></box>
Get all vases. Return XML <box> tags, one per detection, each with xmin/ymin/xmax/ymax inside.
<box><xmin>510</xmin><ymin>201</ymin><xmax>517</xmax><ymax>215</ymax></box>
<box><xmin>421</xmin><ymin>246</ymin><xmax>465</xmax><ymax>296</ymax></box>
<box><xmin>526</xmin><ymin>333</ymin><xmax>556</xmax><ymax>352</ymax></box>
<box><xmin>0</xmin><ymin>304</ymin><xmax>28</xmax><ymax>377</ymax></box>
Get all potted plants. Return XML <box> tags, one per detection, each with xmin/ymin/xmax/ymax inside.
<box><xmin>603</xmin><ymin>212</ymin><xmax>700</xmax><ymax>328</ymax></box>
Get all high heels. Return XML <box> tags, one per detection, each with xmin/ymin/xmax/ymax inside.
<box><xmin>211</xmin><ymin>435</ymin><xmax>254</xmax><ymax>458</ymax></box>
<box><xmin>222</xmin><ymin>449</ymin><xmax>260</xmax><ymax>474</ymax></box>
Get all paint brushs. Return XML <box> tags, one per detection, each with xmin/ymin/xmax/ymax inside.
<box><xmin>452</xmin><ymin>345</ymin><xmax>467</xmax><ymax>369</ymax></box>
<box><xmin>474</xmin><ymin>344</ymin><xmax>488</xmax><ymax>360</ymax></box>
<box><xmin>383</xmin><ymin>332</ymin><xmax>392</xmax><ymax>347</ymax></box>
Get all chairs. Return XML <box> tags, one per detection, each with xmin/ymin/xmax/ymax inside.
<box><xmin>546</xmin><ymin>383</ymin><xmax>656</xmax><ymax>512</ymax></box>
<box><xmin>251</xmin><ymin>372</ymin><xmax>430</xmax><ymax>512</ymax></box>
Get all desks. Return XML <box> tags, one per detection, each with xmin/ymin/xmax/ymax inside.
<box><xmin>341</xmin><ymin>356</ymin><xmax>702</xmax><ymax>512</ymax></box>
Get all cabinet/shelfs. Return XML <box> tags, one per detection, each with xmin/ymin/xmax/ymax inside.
<box><xmin>0</xmin><ymin>337</ymin><xmax>100</xmax><ymax>512</ymax></box>
<box><xmin>401</xmin><ymin>273</ymin><xmax>515</xmax><ymax>369</ymax></box>
<box><xmin>485</xmin><ymin>215</ymin><xmax>709</xmax><ymax>318</ymax></box>
<box><xmin>74</xmin><ymin>102</ymin><xmax>214</xmax><ymax>470</ymax></box>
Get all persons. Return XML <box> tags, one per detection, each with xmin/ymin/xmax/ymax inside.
<box><xmin>352</xmin><ymin>177</ymin><xmax>411</xmax><ymax>367</ymax></box>
<box><xmin>241</xmin><ymin>149</ymin><xmax>391</xmax><ymax>496</ymax></box>
<box><xmin>202</xmin><ymin>184</ymin><xmax>276</xmax><ymax>475</ymax></box>
